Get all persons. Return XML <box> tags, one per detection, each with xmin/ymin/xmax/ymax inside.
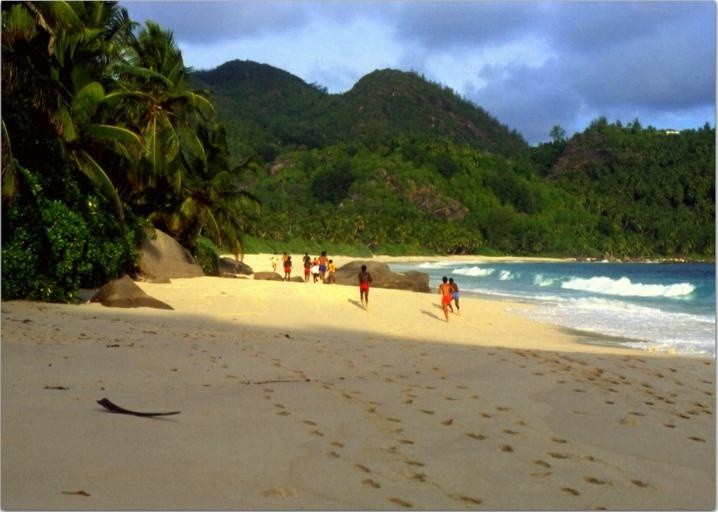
<box><xmin>359</xmin><ymin>265</ymin><xmax>372</xmax><ymax>309</ymax></box>
<box><xmin>449</xmin><ymin>278</ymin><xmax>460</xmax><ymax>313</ymax></box>
<box><xmin>439</xmin><ymin>277</ymin><xmax>454</xmax><ymax>322</ymax></box>
<box><xmin>269</xmin><ymin>251</ymin><xmax>340</xmax><ymax>284</ymax></box>
